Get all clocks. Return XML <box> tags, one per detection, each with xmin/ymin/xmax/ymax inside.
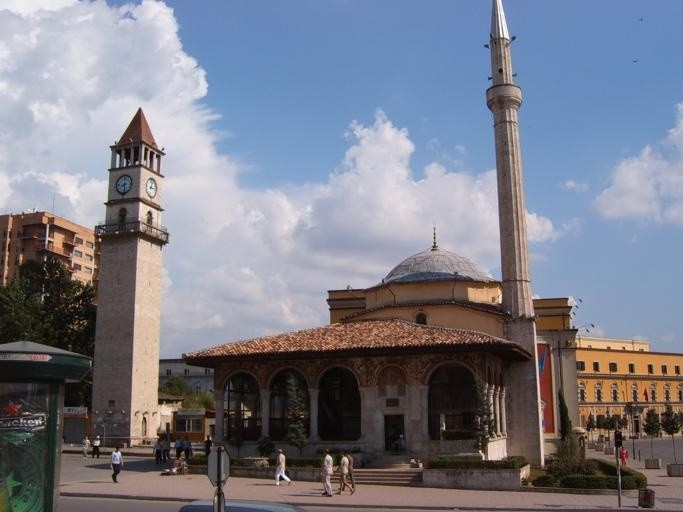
<box><xmin>115</xmin><ymin>174</ymin><xmax>133</xmax><ymax>195</ymax></box>
<box><xmin>145</xmin><ymin>176</ymin><xmax>158</xmax><ymax>198</ymax></box>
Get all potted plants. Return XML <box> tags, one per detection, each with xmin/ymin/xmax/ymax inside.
<box><xmin>642</xmin><ymin>408</ymin><xmax>661</xmax><ymax>469</ymax></box>
<box><xmin>595</xmin><ymin>415</ymin><xmax>605</xmax><ymax>451</ymax></box>
<box><xmin>586</xmin><ymin>411</ymin><xmax>595</xmax><ymax>449</ymax></box>
<box><xmin>659</xmin><ymin>407</ymin><xmax>683</xmax><ymax>477</ymax></box>
<box><xmin>604</xmin><ymin>417</ymin><xmax>614</xmax><ymax>455</ymax></box>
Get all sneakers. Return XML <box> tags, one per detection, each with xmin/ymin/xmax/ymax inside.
<box><xmin>322</xmin><ymin>492</ymin><xmax>333</xmax><ymax>497</ymax></box>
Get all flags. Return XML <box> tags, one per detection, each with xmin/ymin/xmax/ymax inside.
<box><xmin>642</xmin><ymin>388</ymin><xmax>648</xmax><ymax>401</ymax></box>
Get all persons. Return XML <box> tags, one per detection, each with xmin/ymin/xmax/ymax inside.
<box><xmin>318</xmin><ymin>447</ymin><xmax>334</xmax><ymax>497</ymax></box>
<box><xmin>151</xmin><ymin>432</ymin><xmax>193</xmax><ymax>474</ymax></box>
<box><xmin>110</xmin><ymin>444</ymin><xmax>123</xmax><ymax>482</ymax></box>
<box><xmin>91</xmin><ymin>435</ymin><xmax>100</xmax><ymax>458</ymax></box>
<box><xmin>203</xmin><ymin>435</ymin><xmax>211</xmax><ymax>454</ymax></box>
<box><xmin>81</xmin><ymin>435</ymin><xmax>90</xmax><ymax>462</ymax></box>
<box><xmin>335</xmin><ymin>449</ymin><xmax>355</xmax><ymax>494</ymax></box>
<box><xmin>272</xmin><ymin>448</ymin><xmax>291</xmax><ymax>486</ymax></box>
<box><xmin>337</xmin><ymin>448</ymin><xmax>356</xmax><ymax>491</ymax></box>
<box><xmin>619</xmin><ymin>446</ymin><xmax>628</xmax><ymax>466</ymax></box>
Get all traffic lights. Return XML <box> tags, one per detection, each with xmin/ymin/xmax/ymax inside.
<box><xmin>614</xmin><ymin>431</ymin><xmax>623</xmax><ymax>447</ymax></box>
